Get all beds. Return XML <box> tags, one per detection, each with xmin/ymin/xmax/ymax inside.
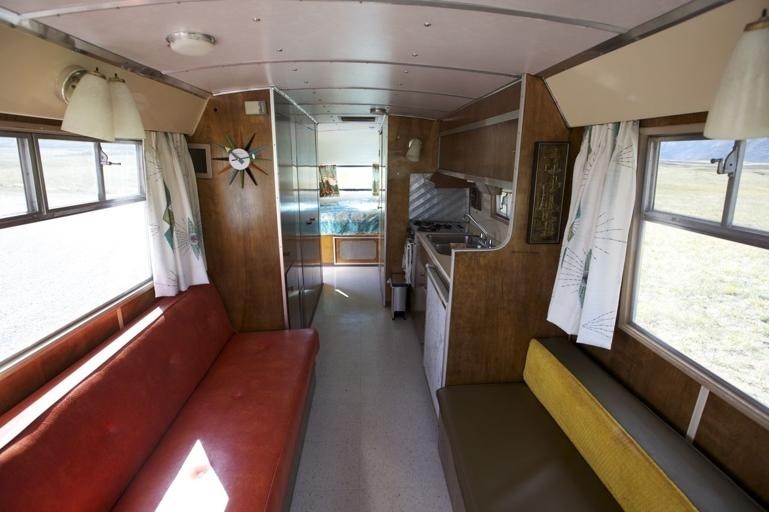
<box><xmin>319</xmin><ymin>198</ymin><xmax>379</xmax><ymax>266</ymax></box>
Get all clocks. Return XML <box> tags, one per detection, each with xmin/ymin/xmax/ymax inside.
<box><xmin>209</xmin><ymin>131</ymin><xmax>272</xmax><ymax>188</ymax></box>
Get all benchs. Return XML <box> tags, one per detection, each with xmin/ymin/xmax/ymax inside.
<box><xmin>435</xmin><ymin>336</ymin><xmax>766</xmax><ymax>512</ymax></box>
<box><xmin>1</xmin><ymin>274</ymin><xmax>320</xmax><ymax>512</ymax></box>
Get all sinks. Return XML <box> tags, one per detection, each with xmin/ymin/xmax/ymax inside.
<box><xmin>435</xmin><ymin>243</ymin><xmax>485</xmax><ymax>254</ymax></box>
<box><xmin>427</xmin><ymin>232</ymin><xmax>474</xmax><ymax>243</ymax></box>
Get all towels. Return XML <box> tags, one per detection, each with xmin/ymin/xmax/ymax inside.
<box><xmin>401</xmin><ymin>240</ymin><xmax>412</xmax><ymax>285</ymax></box>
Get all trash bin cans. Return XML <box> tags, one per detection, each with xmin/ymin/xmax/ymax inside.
<box><xmin>386</xmin><ymin>278</ymin><xmax>410</xmax><ymax>321</ymax></box>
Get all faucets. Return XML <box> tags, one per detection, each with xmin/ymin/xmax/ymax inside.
<box><xmin>463</xmin><ymin>233</ymin><xmax>487</xmax><ymax>244</ymax></box>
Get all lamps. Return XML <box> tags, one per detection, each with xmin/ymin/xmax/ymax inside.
<box><xmin>165</xmin><ymin>31</ymin><xmax>216</xmax><ymax>57</ymax></box>
<box><xmin>55</xmin><ymin>62</ymin><xmax>147</xmax><ymax>143</ymax></box>
<box><xmin>701</xmin><ymin>7</ymin><xmax>769</xmax><ymax>139</ymax></box>
<box><xmin>405</xmin><ymin>136</ymin><xmax>424</xmax><ymax>162</ymax></box>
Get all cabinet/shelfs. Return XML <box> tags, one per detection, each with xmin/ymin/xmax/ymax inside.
<box><xmin>410</xmin><ymin>236</ymin><xmax>428</xmax><ymax>355</ymax></box>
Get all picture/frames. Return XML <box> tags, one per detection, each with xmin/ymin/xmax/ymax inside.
<box><xmin>526</xmin><ymin>141</ymin><xmax>572</xmax><ymax>245</ymax></box>
